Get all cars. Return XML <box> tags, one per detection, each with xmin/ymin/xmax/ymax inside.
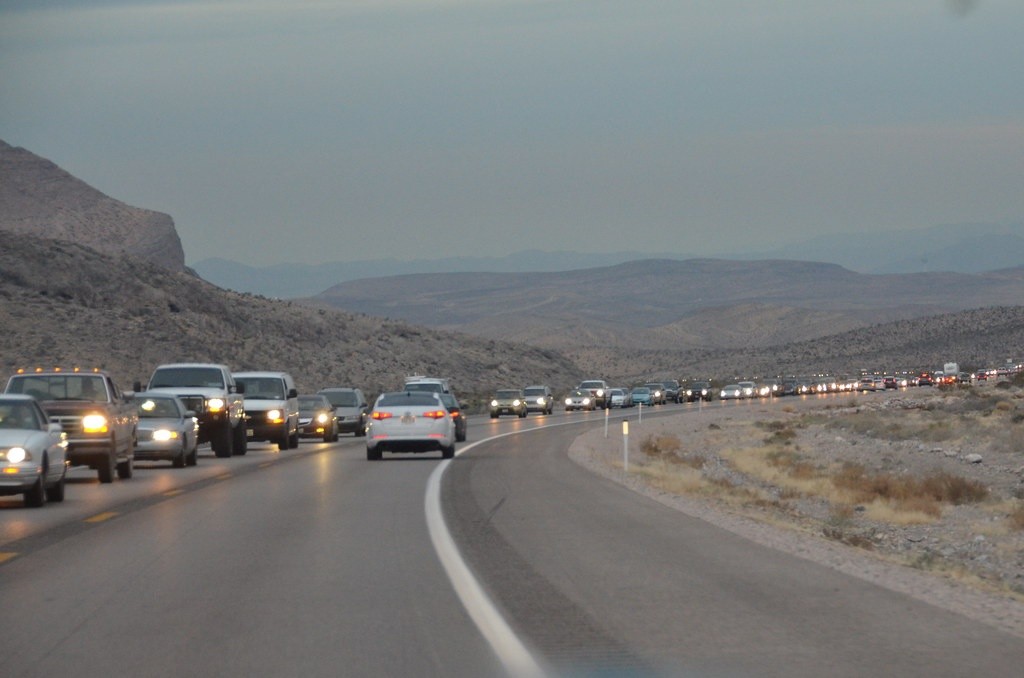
<box><xmin>296</xmin><ymin>393</ymin><xmax>341</xmax><ymax>444</ymax></box>
<box><xmin>564</xmin><ymin>389</ymin><xmax>596</xmax><ymax>411</ymax></box>
<box><xmin>608</xmin><ymin>386</ymin><xmax>633</xmax><ymax>408</ymax></box>
<box><xmin>0</xmin><ymin>393</ymin><xmax>69</xmax><ymax>508</ymax></box>
<box><xmin>440</xmin><ymin>389</ymin><xmax>469</xmax><ymax>442</ymax></box>
<box><xmin>629</xmin><ymin>386</ymin><xmax>655</xmax><ymax>407</ymax></box>
<box><xmin>119</xmin><ymin>391</ymin><xmax>200</xmax><ymax>467</ymax></box>
<box><xmin>719</xmin><ymin>385</ymin><xmax>746</xmax><ymax>400</ymax></box>
<box><xmin>489</xmin><ymin>389</ymin><xmax>529</xmax><ymax>418</ymax></box>
<box><xmin>737</xmin><ymin>364</ymin><xmax>1022</xmax><ymax>400</ymax></box>
<box><xmin>361</xmin><ymin>392</ymin><xmax>459</xmax><ymax>458</ymax></box>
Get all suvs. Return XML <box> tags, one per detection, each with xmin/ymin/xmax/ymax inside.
<box><xmin>686</xmin><ymin>381</ymin><xmax>712</xmax><ymax>402</ymax></box>
<box><xmin>133</xmin><ymin>362</ymin><xmax>248</xmax><ymax>458</ymax></box>
<box><xmin>578</xmin><ymin>381</ymin><xmax>612</xmax><ymax>409</ymax></box>
<box><xmin>311</xmin><ymin>385</ymin><xmax>371</xmax><ymax>437</ymax></box>
<box><xmin>231</xmin><ymin>371</ymin><xmax>301</xmax><ymax>450</ymax></box>
<box><xmin>644</xmin><ymin>383</ymin><xmax>667</xmax><ymax>404</ymax></box>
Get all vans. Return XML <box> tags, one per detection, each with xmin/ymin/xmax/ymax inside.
<box><xmin>402</xmin><ymin>374</ymin><xmax>451</xmax><ymax>392</ymax></box>
<box><xmin>521</xmin><ymin>385</ymin><xmax>553</xmax><ymax>415</ymax></box>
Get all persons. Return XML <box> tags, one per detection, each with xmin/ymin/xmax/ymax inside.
<box><xmin>3</xmin><ymin>405</ymin><xmax>26</xmax><ymax>426</ymax></box>
<box><xmin>78</xmin><ymin>379</ymin><xmax>103</xmax><ymax>400</ymax></box>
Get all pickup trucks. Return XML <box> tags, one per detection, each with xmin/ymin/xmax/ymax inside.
<box><xmin>4</xmin><ymin>366</ymin><xmax>137</xmax><ymax>482</ymax></box>
<box><xmin>660</xmin><ymin>380</ymin><xmax>685</xmax><ymax>404</ymax></box>
